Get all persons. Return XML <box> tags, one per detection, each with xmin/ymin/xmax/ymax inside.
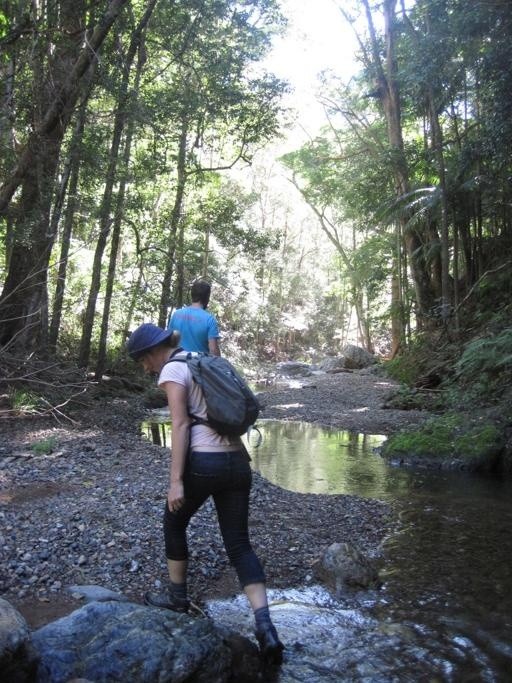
<box><xmin>170</xmin><ymin>281</ymin><xmax>222</xmax><ymax>355</ymax></box>
<box><xmin>125</xmin><ymin>323</ymin><xmax>287</xmax><ymax>659</ymax></box>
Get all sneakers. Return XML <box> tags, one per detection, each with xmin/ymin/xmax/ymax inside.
<box><xmin>144</xmin><ymin>591</ymin><xmax>189</xmax><ymax>612</ymax></box>
<box><xmin>257</xmin><ymin>623</ymin><xmax>284</xmax><ymax>664</ymax></box>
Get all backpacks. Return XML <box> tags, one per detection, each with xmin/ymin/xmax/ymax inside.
<box><xmin>161</xmin><ymin>350</ymin><xmax>260</xmax><ymax>436</ymax></box>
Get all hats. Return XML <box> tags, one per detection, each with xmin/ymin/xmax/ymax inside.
<box><xmin>127</xmin><ymin>324</ymin><xmax>172</xmax><ymax>359</ymax></box>
